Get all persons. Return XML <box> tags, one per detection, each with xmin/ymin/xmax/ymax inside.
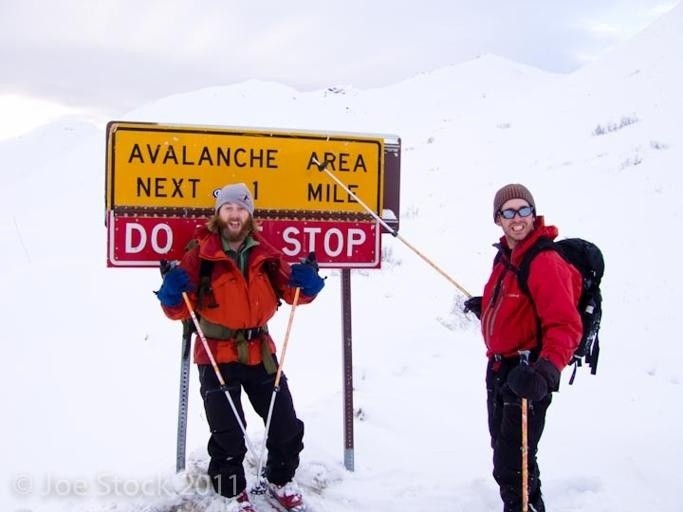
<box><xmin>157</xmin><ymin>181</ymin><xmax>326</xmax><ymax>511</ymax></box>
<box><xmin>462</xmin><ymin>182</ymin><xmax>584</xmax><ymax>512</ymax></box>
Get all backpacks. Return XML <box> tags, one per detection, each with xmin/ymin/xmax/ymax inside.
<box><xmin>518</xmin><ymin>235</ymin><xmax>604</xmax><ymax>384</ymax></box>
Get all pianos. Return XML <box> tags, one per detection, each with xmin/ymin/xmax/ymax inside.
<box><xmin>185</xmin><ymin>431</ymin><xmax>316</xmax><ymax>512</ymax></box>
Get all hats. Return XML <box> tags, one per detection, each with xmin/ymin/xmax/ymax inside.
<box><xmin>493</xmin><ymin>184</ymin><xmax>535</xmax><ymax>223</ymax></box>
<box><xmin>215</xmin><ymin>184</ymin><xmax>254</xmax><ymax>217</ymax></box>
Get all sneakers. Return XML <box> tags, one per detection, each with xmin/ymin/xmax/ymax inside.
<box><xmin>260</xmin><ymin>472</ymin><xmax>302</xmax><ymax>509</ymax></box>
<box><xmin>226</xmin><ymin>489</ymin><xmax>256</xmax><ymax>512</ymax></box>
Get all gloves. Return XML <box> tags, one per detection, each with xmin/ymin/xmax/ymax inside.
<box><xmin>153</xmin><ymin>268</ymin><xmax>194</xmax><ymax>306</ymax></box>
<box><xmin>287</xmin><ymin>264</ymin><xmax>327</xmax><ymax>295</ymax></box>
<box><xmin>463</xmin><ymin>297</ymin><xmax>481</xmax><ymax>319</ymax></box>
<box><xmin>508</xmin><ymin>359</ymin><xmax>560</xmax><ymax>401</ymax></box>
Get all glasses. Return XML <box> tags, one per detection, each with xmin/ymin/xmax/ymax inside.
<box><xmin>500</xmin><ymin>206</ymin><xmax>533</xmax><ymax>219</ymax></box>
